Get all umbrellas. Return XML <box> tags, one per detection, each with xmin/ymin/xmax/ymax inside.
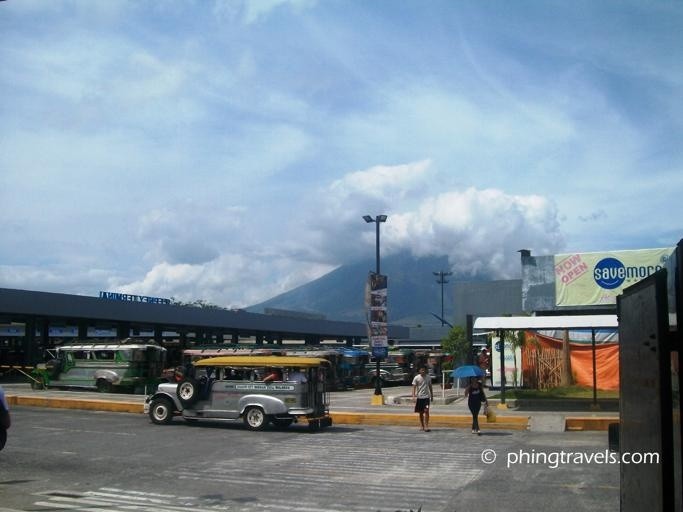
<box><xmin>447</xmin><ymin>364</ymin><xmax>486</xmax><ymax>385</ymax></box>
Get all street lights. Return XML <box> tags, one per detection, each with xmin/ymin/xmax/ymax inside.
<box><xmin>362</xmin><ymin>213</ymin><xmax>389</xmax><ymax>275</ymax></box>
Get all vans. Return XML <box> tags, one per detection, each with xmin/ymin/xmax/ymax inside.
<box><xmin>22</xmin><ymin>337</ymin><xmax>167</xmax><ymax>394</ymax></box>
<box><xmin>158</xmin><ymin>347</ymin><xmax>342</xmax><ymax>394</ymax></box>
<box><xmin>142</xmin><ymin>354</ymin><xmax>334</xmax><ymax>431</ymax></box>
<box><xmin>326</xmin><ymin>341</ymin><xmax>490</xmax><ymax>392</ymax></box>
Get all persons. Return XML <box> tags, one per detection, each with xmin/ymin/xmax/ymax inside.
<box><xmin>410</xmin><ymin>365</ymin><xmax>433</xmax><ymax>432</ymax></box>
<box><xmin>462</xmin><ymin>376</ymin><xmax>487</xmax><ymax>436</ymax></box>
<box><xmin>221</xmin><ymin>368</ymin><xmax>326</xmax><ymax>383</ymax></box>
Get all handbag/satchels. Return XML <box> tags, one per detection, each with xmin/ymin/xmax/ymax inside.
<box><xmin>480</xmin><ymin>391</ymin><xmax>486</xmax><ymax>402</ymax></box>
<box><xmin>483</xmin><ymin>407</ymin><xmax>496</xmax><ymax>423</ymax></box>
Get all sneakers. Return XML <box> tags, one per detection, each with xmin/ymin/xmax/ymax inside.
<box><xmin>472</xmin><ymin>428</ymin><xmax>481</xmax><ymax>434</ymax></box>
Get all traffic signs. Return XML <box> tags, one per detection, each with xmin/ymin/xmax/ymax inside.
<box><xmin>433</xmin><ymin>268</ymin><xmax>454</xmax><ymax>328</ymax></box>
